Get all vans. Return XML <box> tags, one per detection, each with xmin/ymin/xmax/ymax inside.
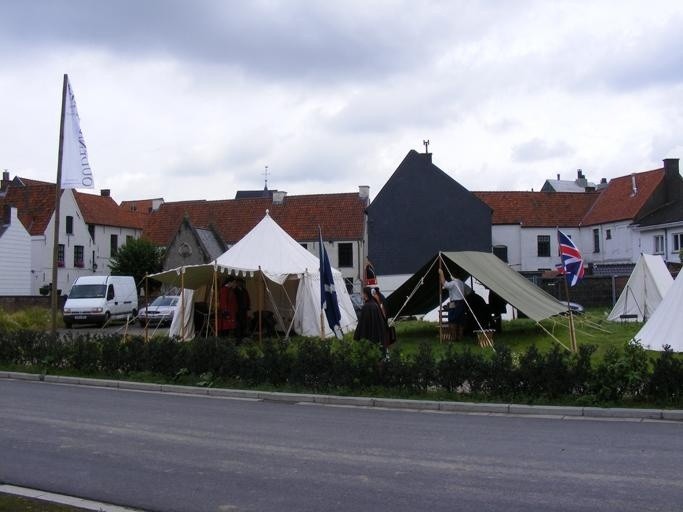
<box><xmin>63</xmin><ymin>275</ymin><xmax>138</xmax><ymax>328</ymax></box>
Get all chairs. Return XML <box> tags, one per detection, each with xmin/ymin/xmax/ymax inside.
<box><xmin>436</xmin><ymin>309</ymin><xmax>457</xmax><ymax>341</ymax></box>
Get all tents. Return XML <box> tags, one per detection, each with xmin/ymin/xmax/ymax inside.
<box><xmin>147</xmin><ymin>207</ymin><xmax>358</xmax><ymax>343</ymax></box>
<box><xmin>627</xmin><ymin>266</ymin><xmax>682</xmax><ymax>352</ymax></box>
<box><xmin>606</xmin><ymin>252</ymin><xmax>674</xmax><ymax>323</ymax></box>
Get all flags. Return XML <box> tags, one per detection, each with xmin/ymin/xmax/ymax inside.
<box><xmin>556</xmin><ymin>229</ymin><xmax>586</xmax><ymax>288</ymax></box>
<box><xmin>60</xmin><ymin>81</ymin><xmax>96</xmax><ymax>192</ymax></box>
<box><xmin>318</xmin><ymin>231</ymin><xmax>341</xmax><ymax>328</ymax></box>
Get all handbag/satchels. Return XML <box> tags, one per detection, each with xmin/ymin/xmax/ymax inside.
<box><xmin>389</xmin><ymin>326</ymin><xmax>397</xmax><ymax>345</ymax></box>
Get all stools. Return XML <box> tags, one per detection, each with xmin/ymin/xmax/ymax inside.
<box><xmin>474</xmin><ymin>329</ymin><xmax>495</xmax><ymax>349</ymax></box>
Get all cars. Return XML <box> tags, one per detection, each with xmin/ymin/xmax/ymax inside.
<box><xmin>138</xmin><ymin>295</ymin><xmax>179</xmax><ymax>326</ymax></box>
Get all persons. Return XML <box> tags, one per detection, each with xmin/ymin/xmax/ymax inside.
<box><xmin>437</xmin><ymin>267</ymin><xmax>508</xmax><ymax>341</ymax></box>
<box><xmin>353</xmin><ymin>287</ymin><xmax>396</xmax><ymax>364</ymax></box>
